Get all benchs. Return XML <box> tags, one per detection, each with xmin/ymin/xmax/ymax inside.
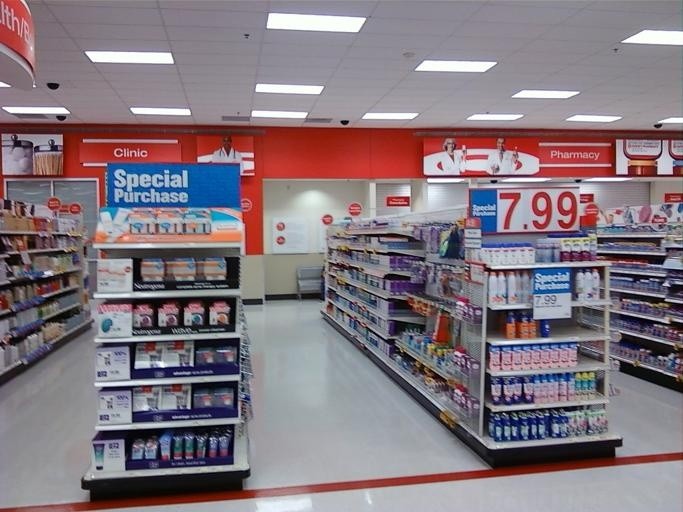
<box><xmin>296</xmin><ymin>266</ymin><xmax>322</xmax><ymax>301</ymax></box>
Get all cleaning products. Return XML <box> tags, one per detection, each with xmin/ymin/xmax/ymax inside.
<box><xmin>487</xmin><ymin>269</ymin><xmax>604</xmax><ymax>306</ymax></box>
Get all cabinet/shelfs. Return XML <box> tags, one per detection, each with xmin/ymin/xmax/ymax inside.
<box><xmin>318</xmin><ymin>215</ymin><xmax>683</xmax><ymax>469</ymax></box>
<box><xmin>0</xmin><ymin>212</ymin><xmax>256</xmax><ymax>501</ymax></box>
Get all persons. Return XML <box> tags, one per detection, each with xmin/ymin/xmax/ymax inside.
<box><xmin>439</xmin><ymin>136</ymin><xmax>467</xmax><ymax>176</ymax></box>
<box><xmin>486</xmin><ymin>137</ymin><xmax>518</xmax><ymax>178</ymax></box>
<box><xmin>211</xmin><ymin>135</ymin><xmax>243</xmax><ymax>178</ymax></box>
